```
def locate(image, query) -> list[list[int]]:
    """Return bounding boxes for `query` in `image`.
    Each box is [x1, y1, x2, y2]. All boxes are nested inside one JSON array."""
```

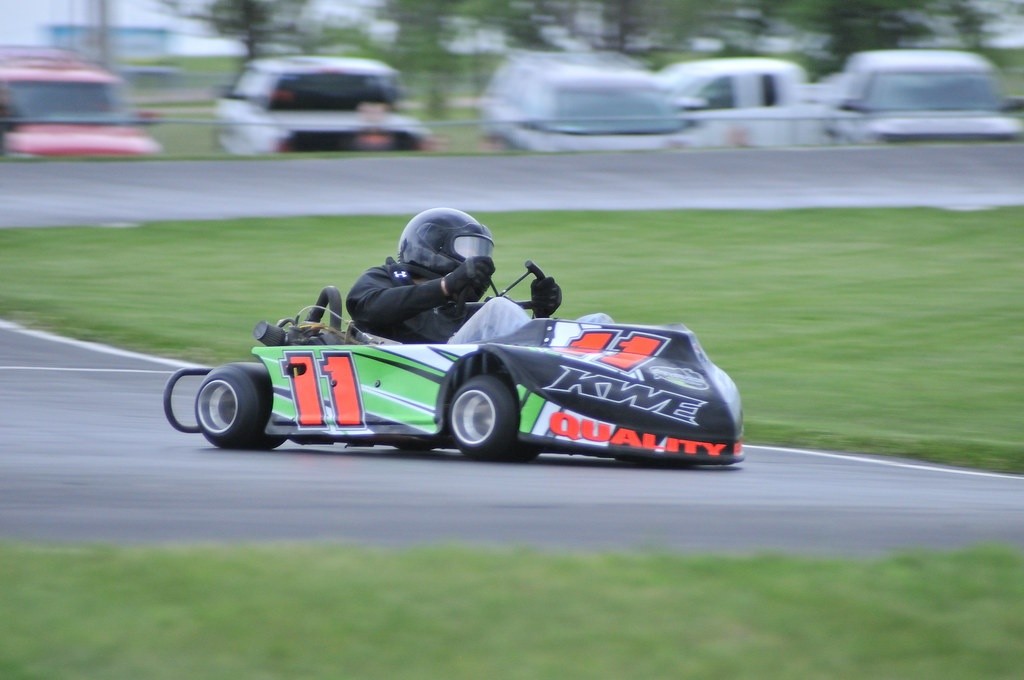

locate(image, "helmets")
[[397, 207, 495, 281]]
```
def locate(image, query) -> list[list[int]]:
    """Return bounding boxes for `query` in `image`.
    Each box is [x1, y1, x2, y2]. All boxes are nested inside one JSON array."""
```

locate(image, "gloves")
[[530, 276, 563, 317], [442, 255, 496, 295]]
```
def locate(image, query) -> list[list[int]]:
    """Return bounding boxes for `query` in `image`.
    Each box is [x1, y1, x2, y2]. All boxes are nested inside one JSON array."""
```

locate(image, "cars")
[[474, 49, 688, 152], [823, 48, 1024, 142]]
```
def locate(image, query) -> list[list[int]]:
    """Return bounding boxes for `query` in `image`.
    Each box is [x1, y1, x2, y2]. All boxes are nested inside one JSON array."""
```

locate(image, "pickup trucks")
[[657, 56, 832, 148], [211, 55, 438, 155]]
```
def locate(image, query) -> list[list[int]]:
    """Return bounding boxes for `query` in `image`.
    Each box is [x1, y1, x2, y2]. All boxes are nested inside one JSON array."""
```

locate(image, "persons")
[[346, 208, 616, 345]]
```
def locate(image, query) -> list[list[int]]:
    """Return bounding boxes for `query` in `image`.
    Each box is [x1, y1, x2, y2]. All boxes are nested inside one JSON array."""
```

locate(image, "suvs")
[[0, 45, 162, 158]]
